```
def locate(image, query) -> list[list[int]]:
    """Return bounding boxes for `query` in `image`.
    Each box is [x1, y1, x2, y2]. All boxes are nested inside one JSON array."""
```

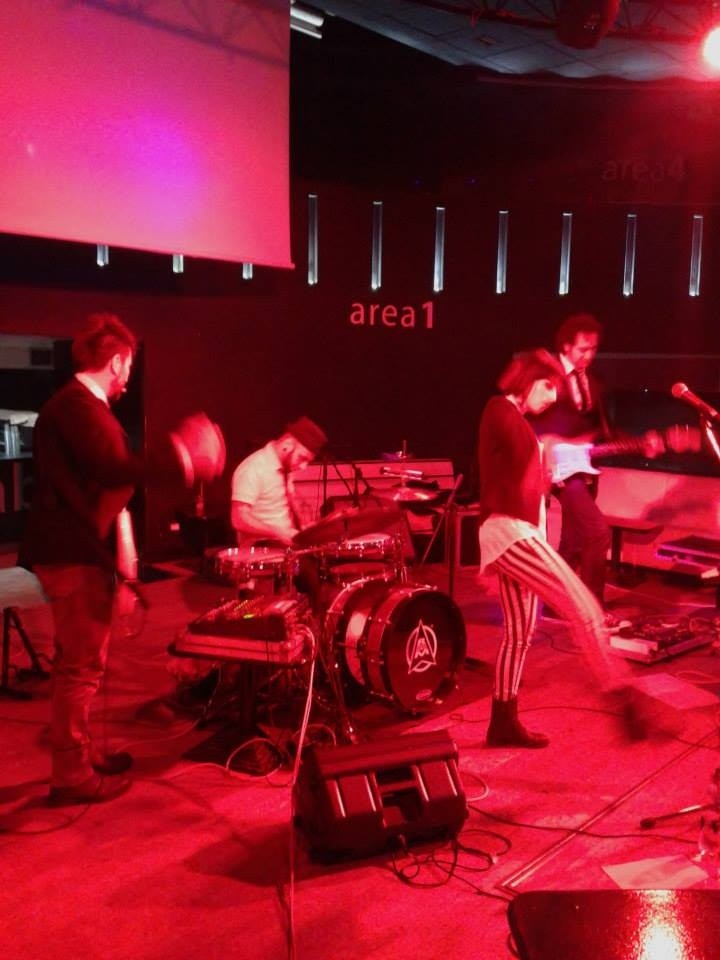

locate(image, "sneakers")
[[92, 752, 132, 776], [600, 613, 633, 633], [48, 770, 130, 805], [539, 605, 569, 625]]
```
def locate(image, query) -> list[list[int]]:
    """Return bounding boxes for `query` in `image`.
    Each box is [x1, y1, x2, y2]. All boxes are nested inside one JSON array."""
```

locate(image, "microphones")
[[671, 382, 720, 424]]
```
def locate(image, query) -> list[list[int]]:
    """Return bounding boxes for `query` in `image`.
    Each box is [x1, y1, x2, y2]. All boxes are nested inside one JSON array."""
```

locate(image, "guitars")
[[114, 510, 138, 617], [542, 424, 687, 483]]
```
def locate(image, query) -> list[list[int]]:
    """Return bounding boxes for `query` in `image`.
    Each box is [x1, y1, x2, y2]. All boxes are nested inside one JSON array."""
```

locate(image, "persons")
[[533, 311, 657, 632], [479, 348, 647, 748], [15, 311, 159, 806], [229, 414, 327, 546]]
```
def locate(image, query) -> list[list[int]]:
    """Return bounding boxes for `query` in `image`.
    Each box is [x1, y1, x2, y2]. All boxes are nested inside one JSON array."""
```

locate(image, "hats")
[[284, 415, 328, 457], [168, 409, 226, 519], [528, 346, 566, 381]]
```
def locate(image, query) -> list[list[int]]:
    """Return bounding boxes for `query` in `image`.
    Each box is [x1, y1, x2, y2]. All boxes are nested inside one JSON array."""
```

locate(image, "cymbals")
[[371, 486, 440, 501], [292, 504, 393, 540]]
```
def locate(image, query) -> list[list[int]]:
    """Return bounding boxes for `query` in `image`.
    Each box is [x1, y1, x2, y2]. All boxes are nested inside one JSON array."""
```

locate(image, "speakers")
[[292, 728, 470, 865]]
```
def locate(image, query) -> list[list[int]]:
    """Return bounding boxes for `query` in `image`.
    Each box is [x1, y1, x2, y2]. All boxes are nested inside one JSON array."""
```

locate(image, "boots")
[[616, 686, 649, 744], [485, 695, 551, 748]]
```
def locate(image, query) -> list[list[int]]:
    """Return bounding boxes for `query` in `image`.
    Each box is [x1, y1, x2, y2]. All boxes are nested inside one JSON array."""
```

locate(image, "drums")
[[316, 533, 396, 556], [325, 577, 465, 714], [327, 560, 397, 595], [216, 547, 294, 595]]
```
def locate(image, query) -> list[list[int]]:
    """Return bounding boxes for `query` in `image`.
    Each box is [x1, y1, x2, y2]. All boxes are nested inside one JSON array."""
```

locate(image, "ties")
[[280, 468, 301, 532], [572, 369, 588, 412]]
